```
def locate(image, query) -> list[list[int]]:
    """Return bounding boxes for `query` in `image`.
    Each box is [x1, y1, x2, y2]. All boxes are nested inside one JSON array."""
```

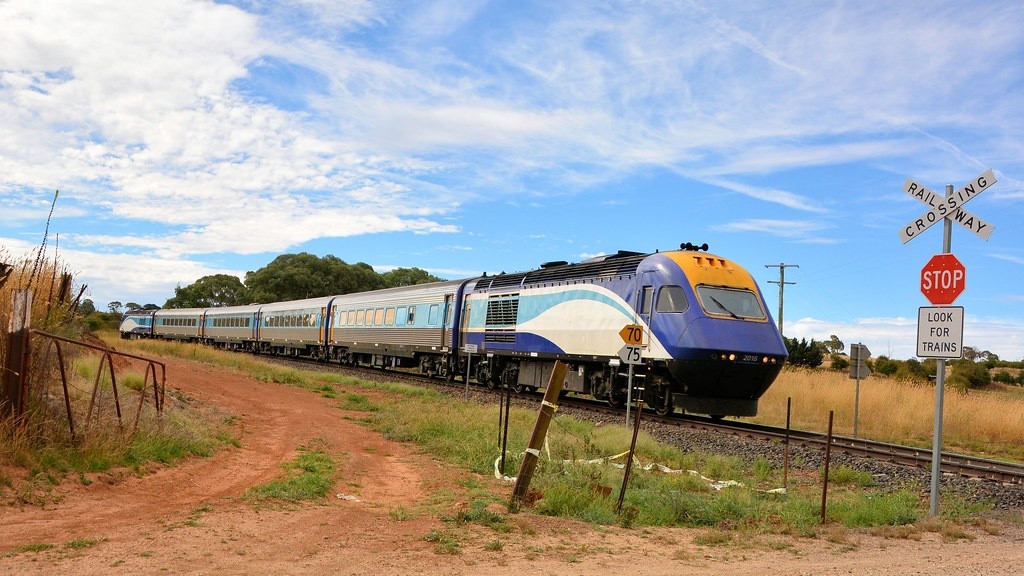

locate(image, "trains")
[[119, 242, 790, 421]]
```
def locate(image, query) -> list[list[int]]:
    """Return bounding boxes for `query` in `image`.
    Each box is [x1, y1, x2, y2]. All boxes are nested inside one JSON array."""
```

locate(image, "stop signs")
[[920, 253, 966, 305]]
